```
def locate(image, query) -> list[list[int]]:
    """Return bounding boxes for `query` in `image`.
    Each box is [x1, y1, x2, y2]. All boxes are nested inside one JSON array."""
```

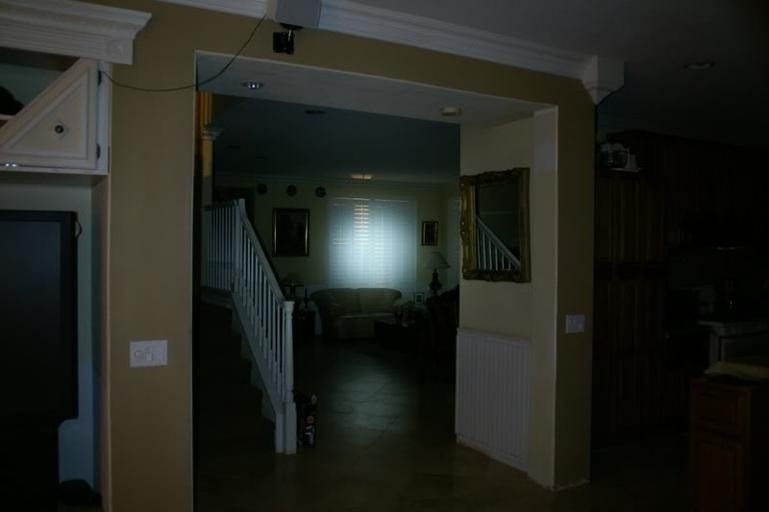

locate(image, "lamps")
[[423, 252, 450, 298]]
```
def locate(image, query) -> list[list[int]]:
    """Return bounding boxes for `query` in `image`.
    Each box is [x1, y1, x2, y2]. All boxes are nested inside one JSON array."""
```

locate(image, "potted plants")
[[294, 392, 320, 448]]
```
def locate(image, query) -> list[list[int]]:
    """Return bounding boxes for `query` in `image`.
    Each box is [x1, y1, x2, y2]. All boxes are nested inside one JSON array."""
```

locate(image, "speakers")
[[272, 1, 323, 31]]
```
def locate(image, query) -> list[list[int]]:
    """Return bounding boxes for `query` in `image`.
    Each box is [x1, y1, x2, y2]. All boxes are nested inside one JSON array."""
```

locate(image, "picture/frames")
[[272, 208, 309, 256], [422, 221, 438, 245]]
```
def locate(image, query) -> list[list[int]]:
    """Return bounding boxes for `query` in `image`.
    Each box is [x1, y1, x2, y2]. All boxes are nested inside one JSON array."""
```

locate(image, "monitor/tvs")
[[1, 207, 80, 418]]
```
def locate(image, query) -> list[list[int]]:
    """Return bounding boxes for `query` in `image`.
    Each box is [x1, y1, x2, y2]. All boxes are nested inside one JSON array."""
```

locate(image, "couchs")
[[311, 288, 401, 344]]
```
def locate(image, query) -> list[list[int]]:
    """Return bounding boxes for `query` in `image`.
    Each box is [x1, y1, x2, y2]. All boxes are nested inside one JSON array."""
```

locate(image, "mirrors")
[[459, 168, 530, 283], [0, 210, 78, 512]]
